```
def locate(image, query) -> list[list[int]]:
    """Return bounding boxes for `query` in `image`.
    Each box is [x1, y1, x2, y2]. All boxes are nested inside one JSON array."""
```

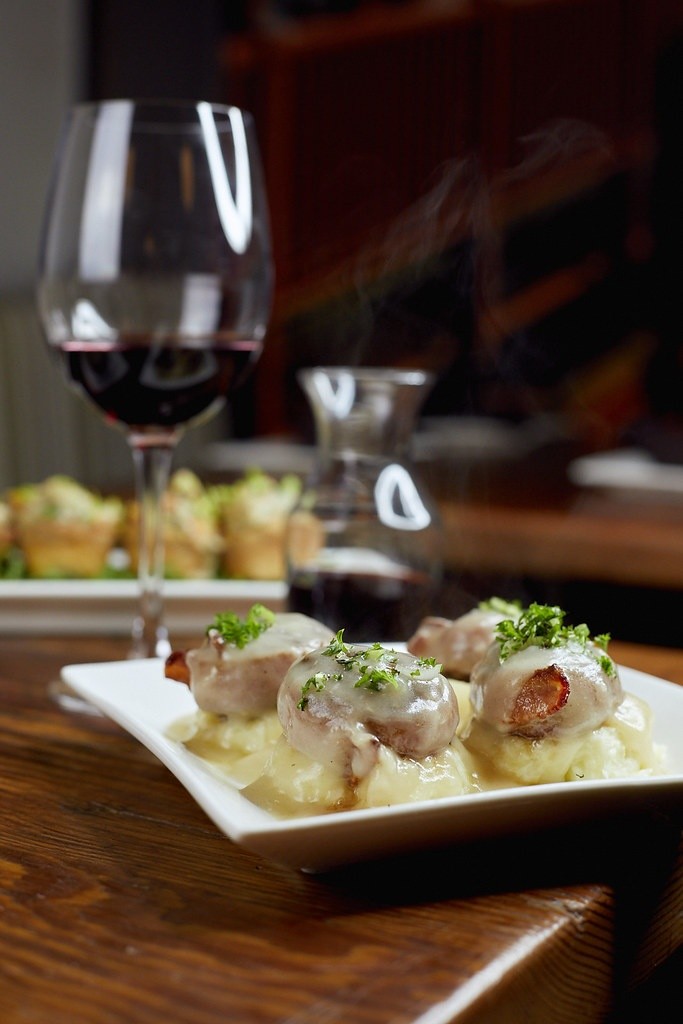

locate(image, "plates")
[[0, 554, 285, 632], [59, 635, 682, 854]]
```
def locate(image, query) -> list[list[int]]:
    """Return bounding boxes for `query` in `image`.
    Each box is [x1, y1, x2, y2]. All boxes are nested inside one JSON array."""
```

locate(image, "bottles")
[[287, 366, 439, 633]]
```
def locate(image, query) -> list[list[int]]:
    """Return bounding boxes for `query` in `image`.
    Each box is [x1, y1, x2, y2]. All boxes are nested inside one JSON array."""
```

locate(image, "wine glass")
[[33, 93, 273, 725]]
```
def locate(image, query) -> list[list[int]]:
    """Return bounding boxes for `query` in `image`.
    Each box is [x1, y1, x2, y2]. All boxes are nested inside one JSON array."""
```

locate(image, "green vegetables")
[[484, 598, 614, 678], [298, 628, 440, 711], [205, 605, 275, 645]]
